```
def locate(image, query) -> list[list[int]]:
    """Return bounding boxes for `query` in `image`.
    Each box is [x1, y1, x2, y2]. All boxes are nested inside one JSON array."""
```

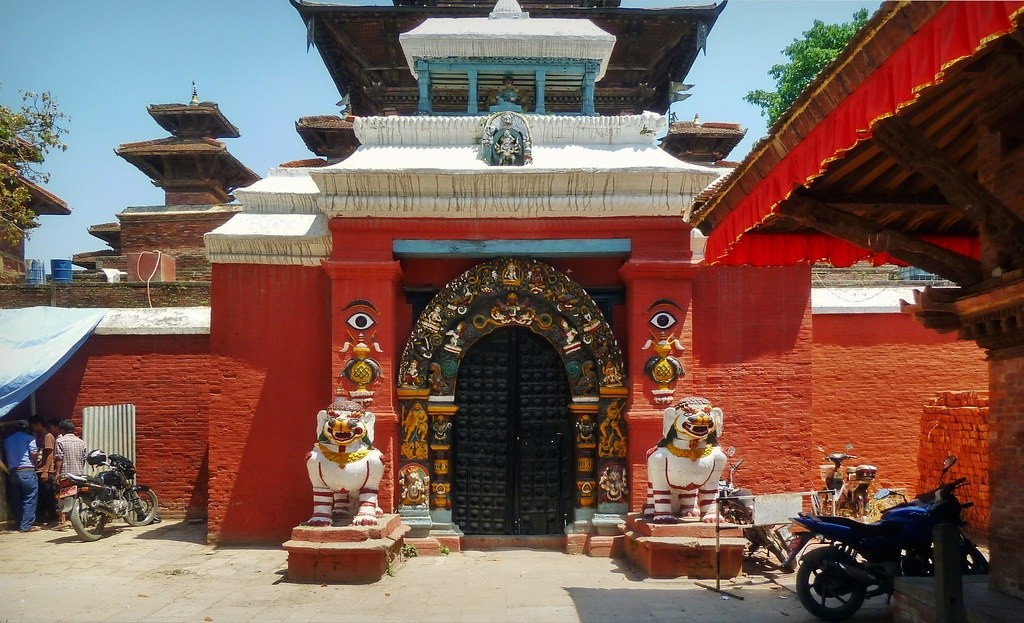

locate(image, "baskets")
[[819, 465, 843, 481]]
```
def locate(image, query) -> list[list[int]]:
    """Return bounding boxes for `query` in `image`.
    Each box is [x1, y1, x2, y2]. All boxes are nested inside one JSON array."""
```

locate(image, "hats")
[[16, 420, 29, 428]]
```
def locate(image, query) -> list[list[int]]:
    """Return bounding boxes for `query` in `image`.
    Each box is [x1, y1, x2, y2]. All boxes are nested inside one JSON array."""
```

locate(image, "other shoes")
[[31, 522, 39, 526], [52, 524, 67, 530], [20, 526, 40, 533]]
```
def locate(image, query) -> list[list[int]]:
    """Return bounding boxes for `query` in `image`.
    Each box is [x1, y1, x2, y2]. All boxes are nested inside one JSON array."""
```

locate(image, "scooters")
[[818, 443, 878, 519]]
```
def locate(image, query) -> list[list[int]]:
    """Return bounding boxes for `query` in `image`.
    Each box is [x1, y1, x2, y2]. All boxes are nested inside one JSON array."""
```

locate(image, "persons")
[[0, 413, 87, 533]]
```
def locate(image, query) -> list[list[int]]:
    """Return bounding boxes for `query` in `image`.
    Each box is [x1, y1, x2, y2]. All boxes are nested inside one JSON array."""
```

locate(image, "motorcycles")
[[59, 449, 159, 542], [719, 445, 798, 572], [792, 455, 990, 623]]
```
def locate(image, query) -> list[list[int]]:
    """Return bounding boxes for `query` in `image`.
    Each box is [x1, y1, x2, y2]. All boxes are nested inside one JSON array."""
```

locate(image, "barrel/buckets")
[[51, 259, 72, 283], [24, 259, 47, 283]]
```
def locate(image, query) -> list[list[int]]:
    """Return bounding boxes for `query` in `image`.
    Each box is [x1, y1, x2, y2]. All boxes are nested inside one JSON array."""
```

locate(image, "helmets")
[[88, 449, 106, 465]]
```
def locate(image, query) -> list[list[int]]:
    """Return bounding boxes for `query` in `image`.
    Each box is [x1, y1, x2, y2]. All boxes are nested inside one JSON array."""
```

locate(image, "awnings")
[[0, 306, 110, 419]]
